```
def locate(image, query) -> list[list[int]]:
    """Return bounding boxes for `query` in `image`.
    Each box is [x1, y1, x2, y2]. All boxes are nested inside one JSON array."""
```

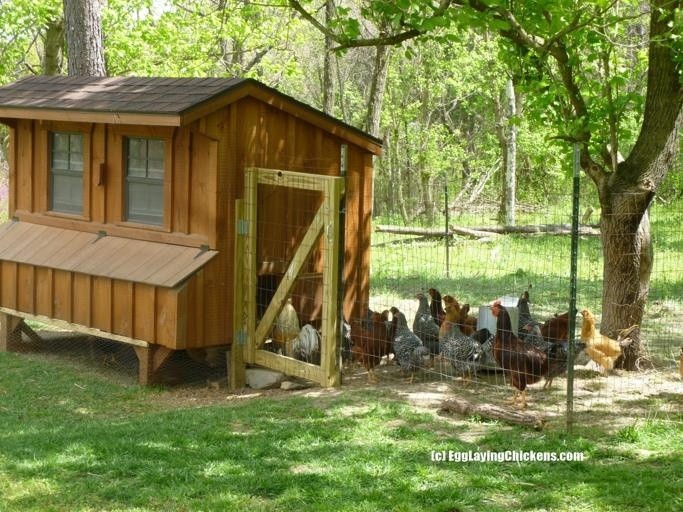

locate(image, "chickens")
[[340, 287, 633, 411]]
